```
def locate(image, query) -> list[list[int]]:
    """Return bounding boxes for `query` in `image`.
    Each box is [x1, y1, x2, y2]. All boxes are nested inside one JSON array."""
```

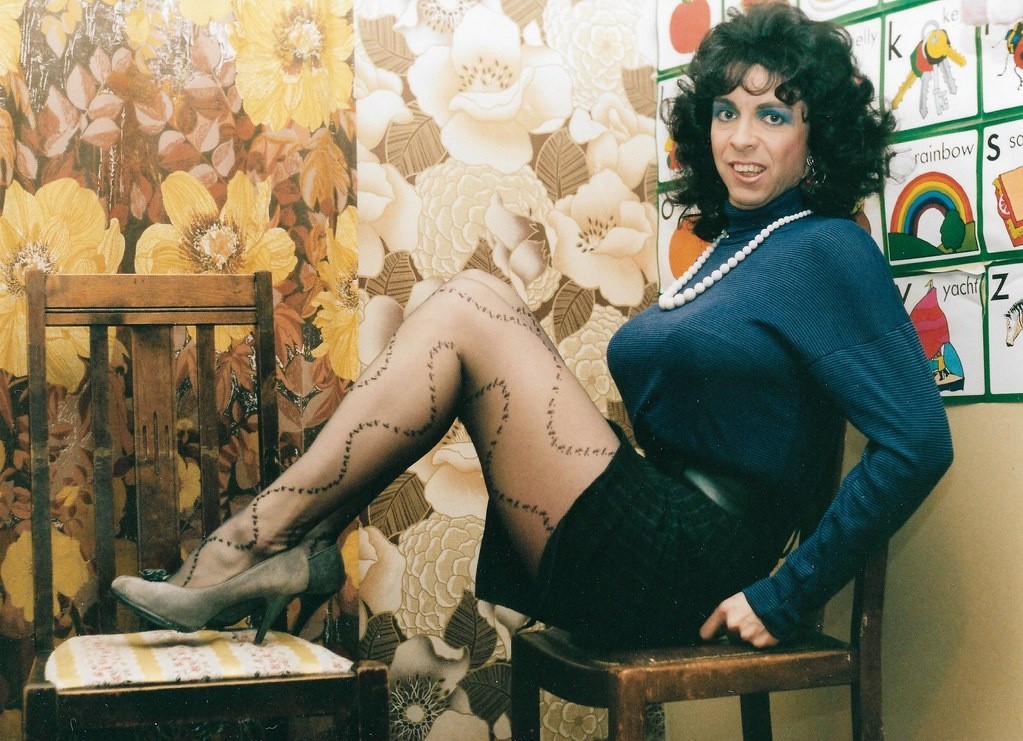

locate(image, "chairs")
[[21, 267, 391, 741], [509, 538, 890, 741]]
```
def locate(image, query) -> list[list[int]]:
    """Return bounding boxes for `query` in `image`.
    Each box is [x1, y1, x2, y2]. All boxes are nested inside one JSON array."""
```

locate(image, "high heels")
[[110, 546, 309, 645], [207, 544, 346, 637]]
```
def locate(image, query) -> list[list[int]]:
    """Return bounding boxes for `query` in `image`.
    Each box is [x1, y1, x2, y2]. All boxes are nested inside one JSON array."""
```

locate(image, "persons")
[[111, 0, 954, 648]]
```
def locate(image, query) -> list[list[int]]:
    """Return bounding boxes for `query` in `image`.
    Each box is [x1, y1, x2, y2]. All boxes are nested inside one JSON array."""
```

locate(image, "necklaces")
[[659, 210, 812, 309]]
